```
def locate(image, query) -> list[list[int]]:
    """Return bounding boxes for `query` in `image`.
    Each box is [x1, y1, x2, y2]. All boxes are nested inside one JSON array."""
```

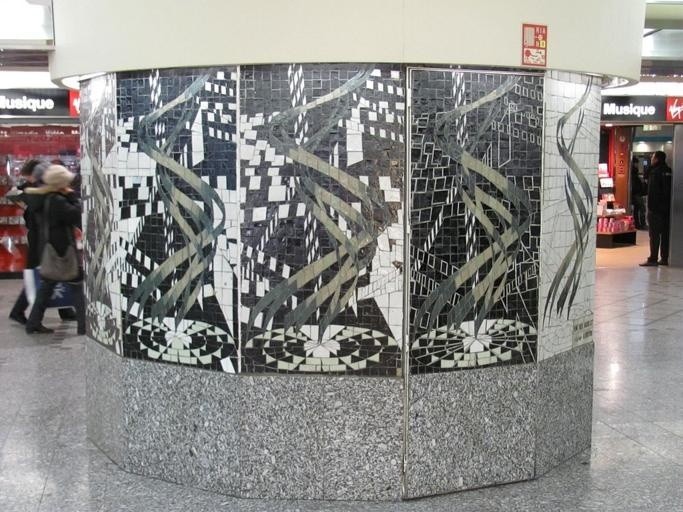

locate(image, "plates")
[[596, 177, 636, 248]]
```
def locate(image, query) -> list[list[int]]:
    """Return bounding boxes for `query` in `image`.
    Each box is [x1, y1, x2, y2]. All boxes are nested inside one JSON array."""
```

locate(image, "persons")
[[9, 160, 83, 334], [631, 155, 647, 230], [639, 151, 673, 266]]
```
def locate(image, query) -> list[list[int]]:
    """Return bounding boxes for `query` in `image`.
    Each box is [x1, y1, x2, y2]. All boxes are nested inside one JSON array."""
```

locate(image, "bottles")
[[41, 164, 81, 188]]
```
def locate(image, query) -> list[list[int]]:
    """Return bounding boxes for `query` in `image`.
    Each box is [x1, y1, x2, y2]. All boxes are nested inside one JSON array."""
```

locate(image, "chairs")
[[639, 256, 669, 267], [9, 309, 27, 325], [24, 323, 55, 334]]
